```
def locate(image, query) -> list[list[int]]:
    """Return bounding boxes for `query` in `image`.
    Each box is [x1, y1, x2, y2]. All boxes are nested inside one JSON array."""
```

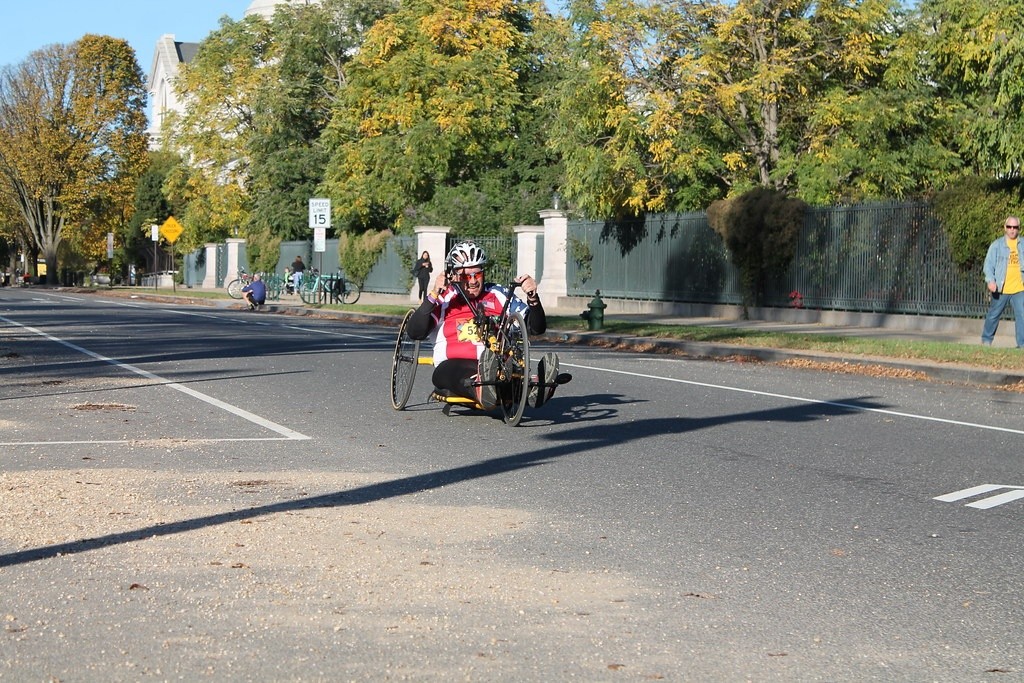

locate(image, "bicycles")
[[227, 266, 282, 301], [298, 266, 362, 305]]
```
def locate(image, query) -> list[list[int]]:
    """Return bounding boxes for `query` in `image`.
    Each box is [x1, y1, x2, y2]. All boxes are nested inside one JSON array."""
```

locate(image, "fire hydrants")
[[577, 288, 608, 331]]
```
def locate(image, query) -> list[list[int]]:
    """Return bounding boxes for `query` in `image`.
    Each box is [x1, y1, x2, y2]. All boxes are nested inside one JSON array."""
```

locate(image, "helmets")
[[444, 240, 487, 269]]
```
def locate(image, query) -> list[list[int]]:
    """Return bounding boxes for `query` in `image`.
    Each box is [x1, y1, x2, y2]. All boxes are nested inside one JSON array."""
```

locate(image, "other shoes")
[[244, 304, 254, 311], [475, 349, 498, 410], [253, 303, 260, 312], [528, 352, 560, 408]]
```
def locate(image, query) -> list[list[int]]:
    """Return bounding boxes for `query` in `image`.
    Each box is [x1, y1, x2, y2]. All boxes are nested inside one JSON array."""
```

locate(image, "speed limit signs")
[[308, 197, 331, 230]]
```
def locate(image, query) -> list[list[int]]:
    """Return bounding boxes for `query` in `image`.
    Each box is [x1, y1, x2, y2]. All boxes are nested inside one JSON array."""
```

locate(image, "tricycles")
[[389, 260, 572, 427]]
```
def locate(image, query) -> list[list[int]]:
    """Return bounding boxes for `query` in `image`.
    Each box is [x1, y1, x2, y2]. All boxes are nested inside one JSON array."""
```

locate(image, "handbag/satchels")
[[410, 260, 421, 277]]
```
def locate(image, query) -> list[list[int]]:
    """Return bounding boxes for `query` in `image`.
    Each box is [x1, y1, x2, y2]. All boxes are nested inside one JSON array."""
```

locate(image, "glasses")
[[1006, 225, 1019, 230], [453, 268, 484, 282]]
